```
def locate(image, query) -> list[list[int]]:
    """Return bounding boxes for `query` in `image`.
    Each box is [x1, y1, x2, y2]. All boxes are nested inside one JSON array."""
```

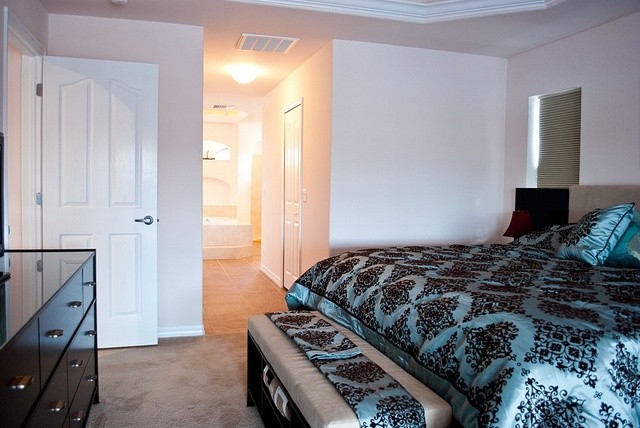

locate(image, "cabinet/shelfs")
[[0, 249, 100, 428]]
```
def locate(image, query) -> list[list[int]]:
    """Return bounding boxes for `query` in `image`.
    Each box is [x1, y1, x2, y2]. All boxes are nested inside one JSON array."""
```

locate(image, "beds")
[[287, 186, 640, 428]]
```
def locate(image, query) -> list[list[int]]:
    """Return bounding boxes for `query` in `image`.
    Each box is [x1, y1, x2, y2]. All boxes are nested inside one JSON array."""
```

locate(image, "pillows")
[[557, 202, 635, 265], [514, 223, 575, 255], [604, 219, 639, 265]]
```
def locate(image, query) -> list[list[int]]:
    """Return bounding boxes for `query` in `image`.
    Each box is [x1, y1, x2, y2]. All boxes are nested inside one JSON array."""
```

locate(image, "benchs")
[[248, 308, 451, 427]]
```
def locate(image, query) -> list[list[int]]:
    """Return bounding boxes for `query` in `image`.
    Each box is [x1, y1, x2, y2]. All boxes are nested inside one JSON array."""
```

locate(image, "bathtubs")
[[203, 217, 253, 259]]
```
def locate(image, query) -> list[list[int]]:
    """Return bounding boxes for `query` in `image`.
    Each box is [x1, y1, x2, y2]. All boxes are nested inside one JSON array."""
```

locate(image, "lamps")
[[504, 210, 534, 242], [227, 63, 262, 86]]
